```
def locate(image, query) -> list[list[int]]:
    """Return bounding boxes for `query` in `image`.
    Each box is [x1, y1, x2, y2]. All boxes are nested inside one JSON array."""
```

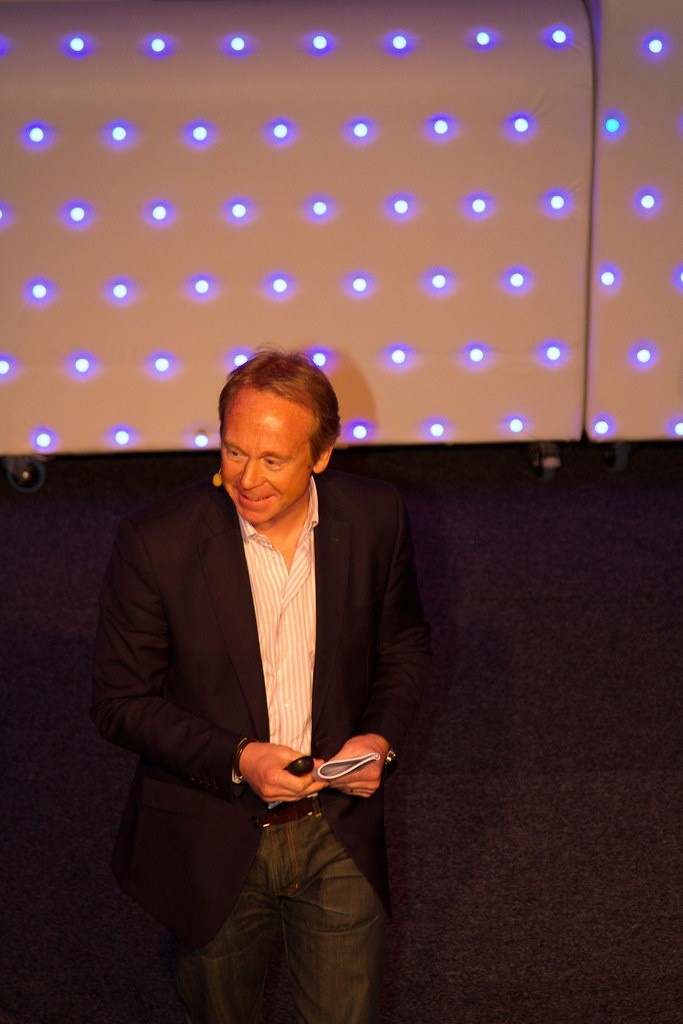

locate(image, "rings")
[[350, 788, 353, 793]]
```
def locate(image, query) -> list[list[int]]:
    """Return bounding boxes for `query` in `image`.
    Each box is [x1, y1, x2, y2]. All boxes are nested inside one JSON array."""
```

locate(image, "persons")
[[91, 353, 427, 1024]]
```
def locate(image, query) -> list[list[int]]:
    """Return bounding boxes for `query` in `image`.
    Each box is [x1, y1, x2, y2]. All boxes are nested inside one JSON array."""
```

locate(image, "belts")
[[261, 794, 320, 827]]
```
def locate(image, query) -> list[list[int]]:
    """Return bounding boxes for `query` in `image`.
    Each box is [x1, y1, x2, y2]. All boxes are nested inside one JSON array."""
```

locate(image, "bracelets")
[[234, 738, 259, 782]]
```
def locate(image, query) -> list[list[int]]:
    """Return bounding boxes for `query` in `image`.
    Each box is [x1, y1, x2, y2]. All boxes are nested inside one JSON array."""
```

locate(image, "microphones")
[[213, 469, 223, 487]]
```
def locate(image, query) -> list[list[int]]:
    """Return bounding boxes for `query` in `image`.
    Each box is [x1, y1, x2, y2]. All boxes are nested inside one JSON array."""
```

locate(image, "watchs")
[[384, 748, 396, 766]]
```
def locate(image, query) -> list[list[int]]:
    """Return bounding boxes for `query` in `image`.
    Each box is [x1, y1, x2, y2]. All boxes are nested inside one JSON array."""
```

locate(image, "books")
[[313, 753, 378, 781]]
